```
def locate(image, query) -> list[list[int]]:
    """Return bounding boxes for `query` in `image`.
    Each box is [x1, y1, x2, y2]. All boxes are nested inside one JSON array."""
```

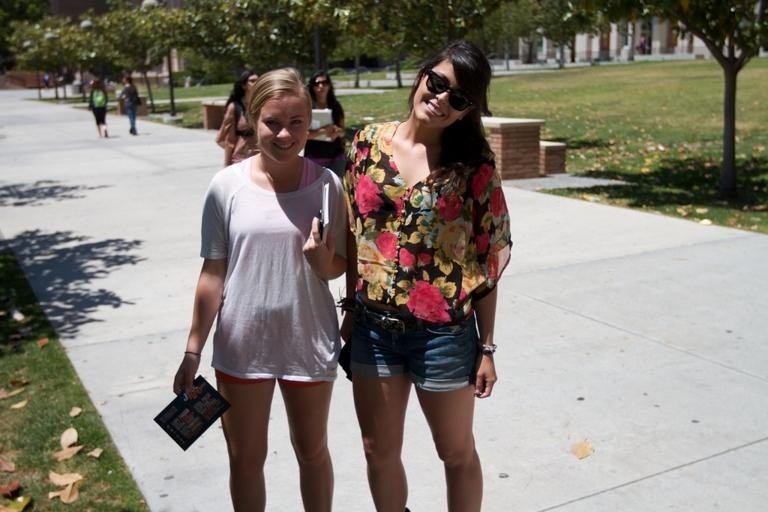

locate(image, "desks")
[[480, 116, 545, 181], [117, 96, 147, 116], [201, 101, 228, 129]]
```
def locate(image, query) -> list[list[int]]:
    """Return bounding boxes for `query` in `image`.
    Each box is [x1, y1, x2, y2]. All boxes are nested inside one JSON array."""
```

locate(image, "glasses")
[[422, 66, 476, 111], [313, 79, 329, 87]]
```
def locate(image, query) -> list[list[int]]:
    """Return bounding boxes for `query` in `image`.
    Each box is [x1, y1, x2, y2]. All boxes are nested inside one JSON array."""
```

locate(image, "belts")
[[352, 298, 417, 334]]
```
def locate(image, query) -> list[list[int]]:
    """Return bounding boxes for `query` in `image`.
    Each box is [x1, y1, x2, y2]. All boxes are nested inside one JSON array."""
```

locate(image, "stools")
[[540, 141, 567, 175]]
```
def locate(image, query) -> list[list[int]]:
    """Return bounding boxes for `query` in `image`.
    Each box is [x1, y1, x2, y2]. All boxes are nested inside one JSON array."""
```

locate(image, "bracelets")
[[183, 350, 203, 357], [477, 340, 497, 355], [337, 297, 357, 314]]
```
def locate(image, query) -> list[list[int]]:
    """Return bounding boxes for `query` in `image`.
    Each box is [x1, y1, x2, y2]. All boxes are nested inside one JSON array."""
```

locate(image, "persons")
[[88, 79, 108, 138], [335, 38, 514, 511], [116, 75, 138, 135], [639, 33, 647, 55], [303, 70, 349, 181], [171, 66, 351, 510], [214, 68, 263, 171]]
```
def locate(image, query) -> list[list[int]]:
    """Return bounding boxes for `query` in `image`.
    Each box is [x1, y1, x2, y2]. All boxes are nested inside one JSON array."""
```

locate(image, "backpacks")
[[93, 89, 107, 108], [128, 89, 141, 106]]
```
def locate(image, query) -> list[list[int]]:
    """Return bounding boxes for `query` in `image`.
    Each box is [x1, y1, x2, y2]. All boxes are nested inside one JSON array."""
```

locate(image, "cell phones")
[[319, 208, 324, 240]]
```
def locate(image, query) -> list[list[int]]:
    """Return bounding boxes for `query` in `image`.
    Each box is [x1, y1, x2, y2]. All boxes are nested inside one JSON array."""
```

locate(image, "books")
[[320, 180, 332, 245], [308, 108, 332, 143]]
